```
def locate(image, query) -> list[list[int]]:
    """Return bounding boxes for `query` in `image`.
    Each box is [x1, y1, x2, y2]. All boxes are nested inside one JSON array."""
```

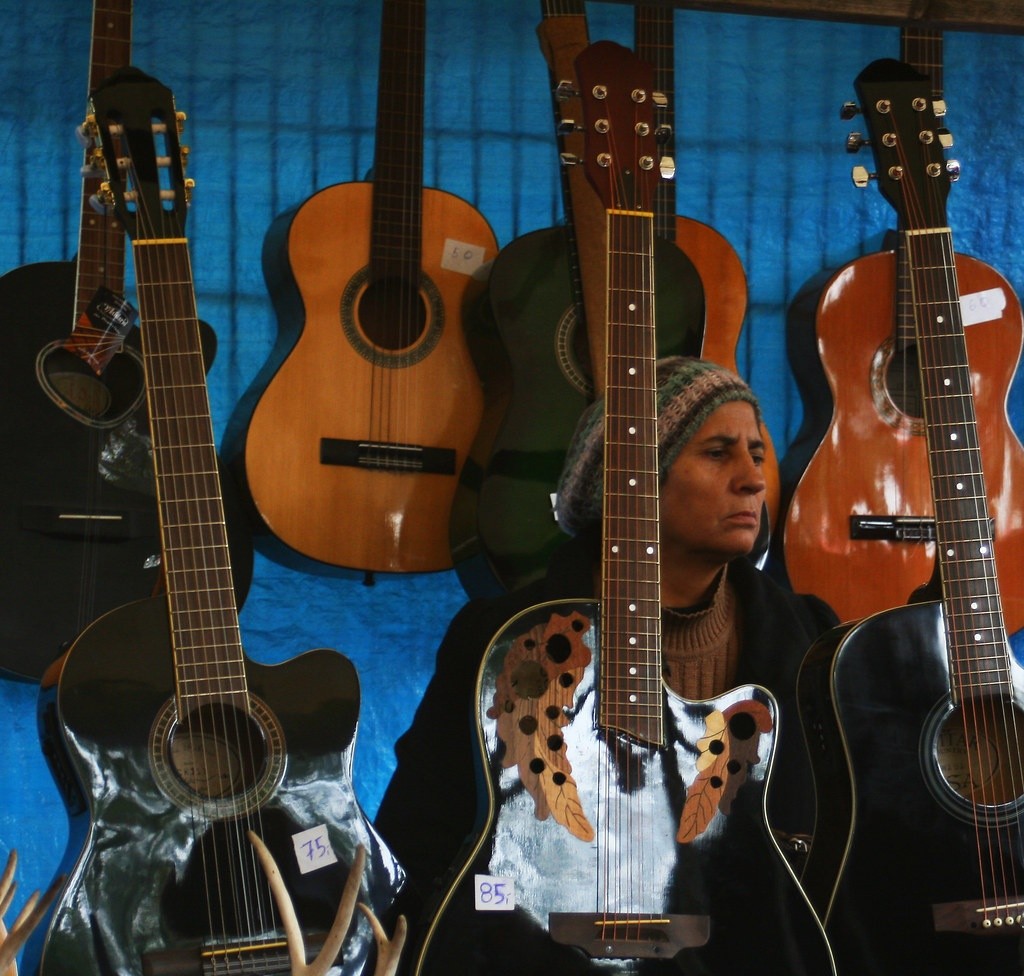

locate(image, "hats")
[[555, 356, 761, 537]]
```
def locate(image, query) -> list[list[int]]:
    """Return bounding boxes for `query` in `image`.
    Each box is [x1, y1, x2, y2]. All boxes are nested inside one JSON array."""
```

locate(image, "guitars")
[[792, 56, 1024, 975], [373, 42, 841, 976], [768, 1, 1024, 641], [635, 1, 782, 545], [0, 0, 255, 687], [219, 2, 506, 585], [20, 64, 371, 976], [445, 1, 710, 599]]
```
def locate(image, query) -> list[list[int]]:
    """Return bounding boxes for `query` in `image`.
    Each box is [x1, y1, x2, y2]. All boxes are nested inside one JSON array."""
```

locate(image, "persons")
[[372, 357, 839, 976]]
